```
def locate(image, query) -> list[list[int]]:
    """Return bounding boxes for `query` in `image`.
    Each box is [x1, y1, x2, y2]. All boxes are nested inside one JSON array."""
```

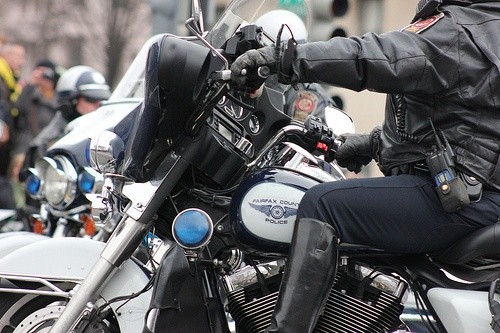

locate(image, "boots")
[[263, 218, 341, 333]]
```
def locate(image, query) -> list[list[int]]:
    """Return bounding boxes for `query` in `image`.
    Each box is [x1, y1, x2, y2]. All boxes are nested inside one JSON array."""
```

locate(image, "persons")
[[230, 0, 500, 333], [0, 52, 112, 232]]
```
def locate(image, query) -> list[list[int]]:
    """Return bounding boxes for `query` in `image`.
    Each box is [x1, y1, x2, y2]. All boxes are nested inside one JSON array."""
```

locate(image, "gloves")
[[230, 40, 284, 94], [323, 127, 378, 174]]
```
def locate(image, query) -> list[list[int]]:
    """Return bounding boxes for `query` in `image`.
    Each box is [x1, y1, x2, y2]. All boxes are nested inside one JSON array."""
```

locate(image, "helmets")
[[56, 64, 110, 106]]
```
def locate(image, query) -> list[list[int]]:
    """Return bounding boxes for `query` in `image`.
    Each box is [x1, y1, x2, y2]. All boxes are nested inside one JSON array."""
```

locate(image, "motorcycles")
[[1, 0, 499, 333]]
